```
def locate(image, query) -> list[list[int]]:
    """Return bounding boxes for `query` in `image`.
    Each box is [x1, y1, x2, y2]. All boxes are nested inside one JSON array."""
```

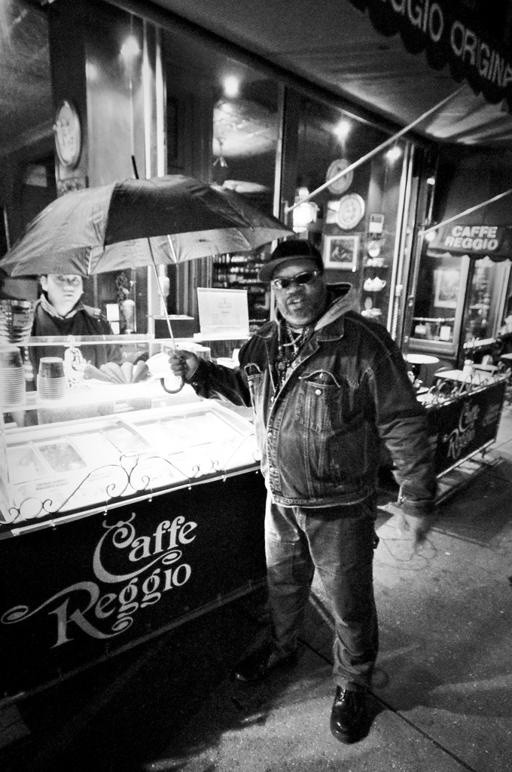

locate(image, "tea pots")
[[364, 276, 386, 291]]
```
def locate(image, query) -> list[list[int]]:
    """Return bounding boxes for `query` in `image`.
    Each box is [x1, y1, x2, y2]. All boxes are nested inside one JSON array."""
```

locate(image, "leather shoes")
[[330, 684, 363, 744], [231, 641, 299, 685]]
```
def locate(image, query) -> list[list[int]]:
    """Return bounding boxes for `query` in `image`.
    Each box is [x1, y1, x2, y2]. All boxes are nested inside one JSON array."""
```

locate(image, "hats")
[[257, 239, 322, 280]]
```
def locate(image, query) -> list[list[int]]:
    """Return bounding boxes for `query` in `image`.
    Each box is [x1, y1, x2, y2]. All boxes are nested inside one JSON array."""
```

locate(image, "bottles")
[[462, 359, 474, 380], [1, 299, 33, 346]]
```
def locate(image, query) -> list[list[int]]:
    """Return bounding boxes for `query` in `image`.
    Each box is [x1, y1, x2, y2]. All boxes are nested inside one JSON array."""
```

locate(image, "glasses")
[[270, 270, 323, 289]]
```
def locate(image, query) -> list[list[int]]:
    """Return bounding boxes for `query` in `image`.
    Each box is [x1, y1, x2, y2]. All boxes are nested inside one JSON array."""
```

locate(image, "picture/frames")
[[321, 234, 360, 273]]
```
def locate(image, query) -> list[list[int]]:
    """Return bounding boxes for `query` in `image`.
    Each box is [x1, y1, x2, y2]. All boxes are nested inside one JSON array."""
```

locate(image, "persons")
[[165, 237, 436, 744], [32, 270, 121, 370]]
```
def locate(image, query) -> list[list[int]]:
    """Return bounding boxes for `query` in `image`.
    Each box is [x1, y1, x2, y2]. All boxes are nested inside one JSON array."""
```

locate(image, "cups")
[[439, 326, 451, 341], [415, 325, 426, 339], [1, 347, 65, 406]]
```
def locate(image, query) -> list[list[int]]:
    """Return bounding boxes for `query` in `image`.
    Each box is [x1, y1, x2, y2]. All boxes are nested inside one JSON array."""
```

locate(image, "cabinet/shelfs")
[[0, 332, 269, 771], [205, 237, 273, 328]]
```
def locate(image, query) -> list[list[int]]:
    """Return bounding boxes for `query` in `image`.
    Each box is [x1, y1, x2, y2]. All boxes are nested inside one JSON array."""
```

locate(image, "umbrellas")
[[1, 152, 297, 394]]
